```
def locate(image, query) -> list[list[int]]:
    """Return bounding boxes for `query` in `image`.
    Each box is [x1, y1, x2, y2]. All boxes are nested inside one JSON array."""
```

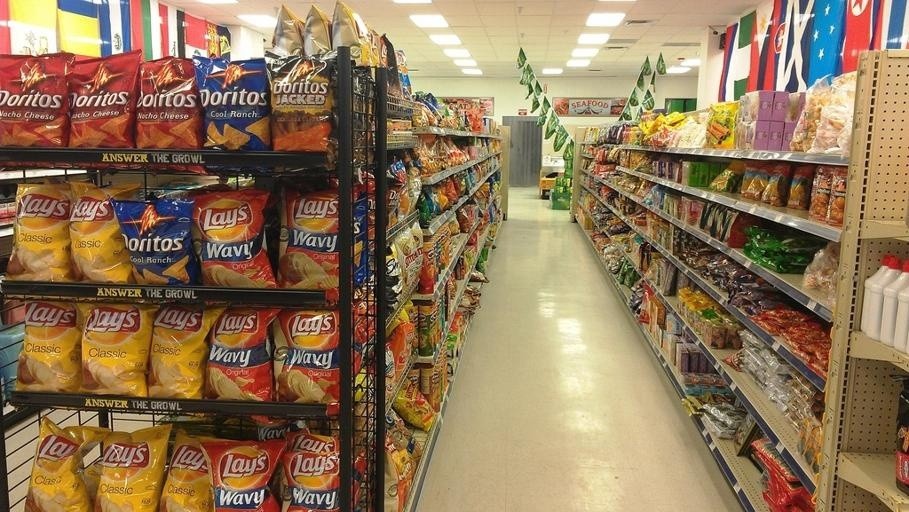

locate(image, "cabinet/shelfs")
[[540, 154, 565, 200], [380, 101, 506, 511], [816, 51, 905, 512], [575, 55, 853, 512], [0, 50, 385, 512]]
